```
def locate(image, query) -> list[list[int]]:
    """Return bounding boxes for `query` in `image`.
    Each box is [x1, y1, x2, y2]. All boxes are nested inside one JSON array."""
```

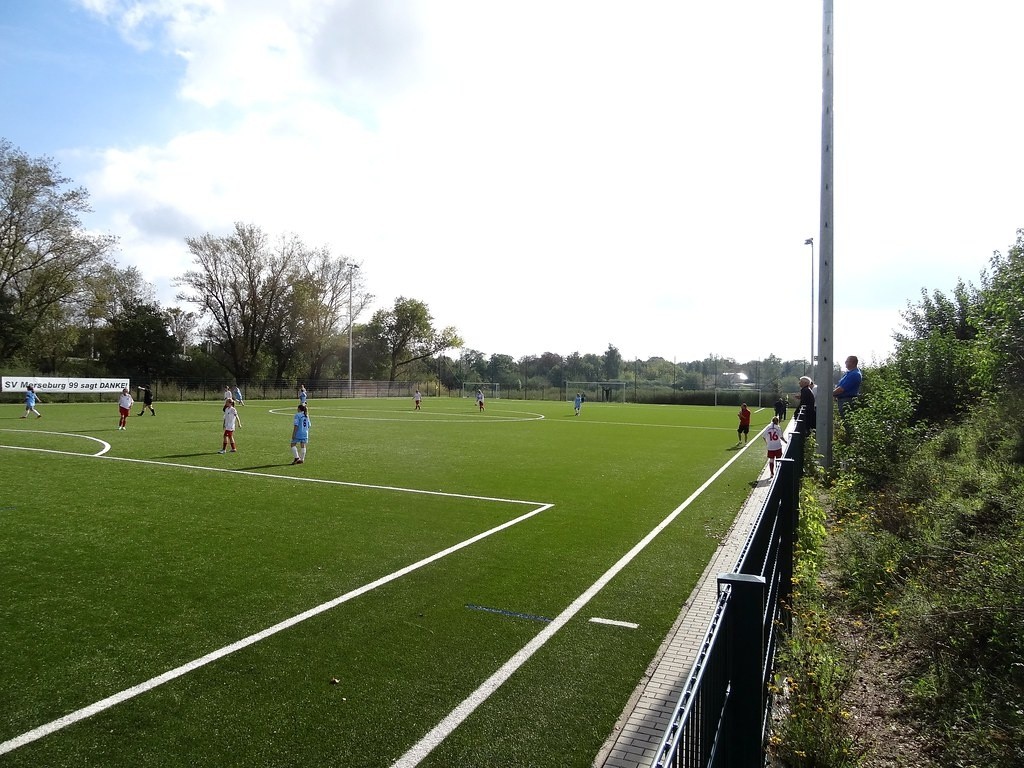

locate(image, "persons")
[[475, 389, 485, 413], [298, 385, 308, 407], [581, 392, 585, 402], [794, 376, 817, 437], [118, 388, 134, 431], [137, 385, 156, 416], [291, 405, 311, 465], [574, 393, 581, 416], [412, 389, 422, 410], [603, 388, 610, 403], [19, 386, 42, 419], [833, 356, 862, 418], [218, 399, 241, 454], [762, 416, 787, 479], [222, 385, 244, 412], [773, 397, 789, 423], [736, 403, 751, 446]]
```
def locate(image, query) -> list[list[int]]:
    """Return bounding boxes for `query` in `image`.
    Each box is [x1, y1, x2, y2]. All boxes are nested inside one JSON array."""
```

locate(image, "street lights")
[[805, 237, 814, 382], [347, 263, 359, 395]]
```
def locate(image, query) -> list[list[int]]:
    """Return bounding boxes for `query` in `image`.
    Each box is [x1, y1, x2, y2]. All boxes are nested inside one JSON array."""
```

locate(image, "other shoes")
[[37, 415, 41, 418], [137, 414, 143, 416], [20, 416, 26, 418], [151, 414, 156, 416], [118, 426, 126, 430], [736, 442, 747, 447], [292, 458, 303, 464]]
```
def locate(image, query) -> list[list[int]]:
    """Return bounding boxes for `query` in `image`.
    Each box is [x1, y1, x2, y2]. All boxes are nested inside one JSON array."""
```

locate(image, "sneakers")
[[229, 449, 236, 452], [218, 449, 226, 454]]
